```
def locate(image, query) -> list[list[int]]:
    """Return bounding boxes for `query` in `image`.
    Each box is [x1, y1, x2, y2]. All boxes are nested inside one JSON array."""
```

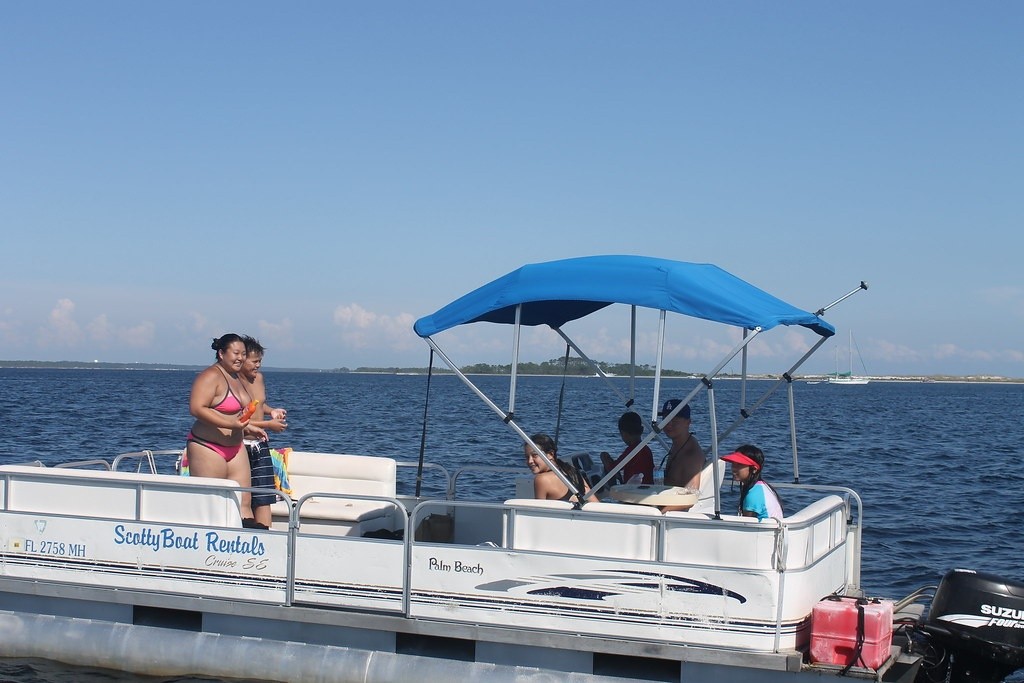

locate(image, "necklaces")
[[664, 433, 693, 478]]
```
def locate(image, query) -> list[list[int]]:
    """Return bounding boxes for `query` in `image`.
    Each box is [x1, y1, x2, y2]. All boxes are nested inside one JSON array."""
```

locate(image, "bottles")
[[237, 400, 259, 422], [653, 464, 665, 490], [627, 473, 644, 490]]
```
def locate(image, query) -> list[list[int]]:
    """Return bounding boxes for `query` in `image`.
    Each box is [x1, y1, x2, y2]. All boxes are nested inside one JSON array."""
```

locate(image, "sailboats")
[[826, 329, 870, 385]]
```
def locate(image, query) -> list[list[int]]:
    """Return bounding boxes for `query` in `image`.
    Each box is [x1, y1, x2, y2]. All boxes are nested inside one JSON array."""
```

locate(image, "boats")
[[806, 381, 821, 385], [0, 255, 1024, 683], [595, 372, 617, 378]]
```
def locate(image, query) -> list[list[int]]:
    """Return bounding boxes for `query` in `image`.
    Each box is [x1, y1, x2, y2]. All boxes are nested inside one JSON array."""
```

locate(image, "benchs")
[[271, 451, 397, 537]]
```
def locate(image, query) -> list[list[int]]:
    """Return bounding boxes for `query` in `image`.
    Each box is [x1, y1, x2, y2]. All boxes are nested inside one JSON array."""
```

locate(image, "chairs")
[[681, 458, 726, 513]]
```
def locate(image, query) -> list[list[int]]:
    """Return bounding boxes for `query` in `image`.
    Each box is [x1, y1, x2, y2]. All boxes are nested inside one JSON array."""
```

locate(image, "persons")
[[608, 412, 654, 485], [187, 334, 288, 530], [525, 433, 600, 502], [719, 445, 783, 521], [658, 399, 705, 514]]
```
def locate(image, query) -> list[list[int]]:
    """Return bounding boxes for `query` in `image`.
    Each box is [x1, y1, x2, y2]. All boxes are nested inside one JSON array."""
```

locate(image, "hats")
[[719, 451, 760, 471], [657, 399, 690, 418]]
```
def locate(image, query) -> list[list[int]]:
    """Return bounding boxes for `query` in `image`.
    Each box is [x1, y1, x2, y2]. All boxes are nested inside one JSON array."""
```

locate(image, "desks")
[[608, 482, 698, 509]]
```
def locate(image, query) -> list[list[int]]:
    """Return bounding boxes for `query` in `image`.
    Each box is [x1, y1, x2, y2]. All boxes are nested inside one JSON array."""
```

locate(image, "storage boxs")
[[809, 598, 895, 669]]
